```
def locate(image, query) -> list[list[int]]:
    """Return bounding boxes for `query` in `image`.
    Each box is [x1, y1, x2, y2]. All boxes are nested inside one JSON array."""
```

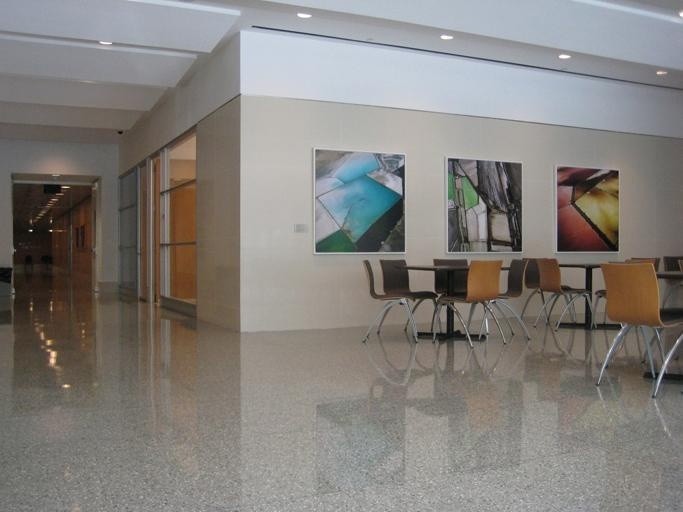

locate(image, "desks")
[[397, 261, 513, 343], [554, 259, 620, 332], [633, 266, 681, 383]]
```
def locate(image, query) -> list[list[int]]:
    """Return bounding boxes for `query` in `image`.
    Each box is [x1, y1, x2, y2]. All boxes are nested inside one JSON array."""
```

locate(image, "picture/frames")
[[444, 155, 523, 255], [553, 164, 620, 254], [313, 147, 407, 255]]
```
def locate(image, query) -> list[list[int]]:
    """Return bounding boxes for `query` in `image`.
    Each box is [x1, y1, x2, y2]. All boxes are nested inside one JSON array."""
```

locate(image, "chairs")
[[430, 259, 476, 339], [363, 260, 440, 348], [533, 258, 597, 336], [478, 259, 535, 343], [453, 259, 508, 350], [592, 262, 680, 398], [521, 258, 572, 326], [377, 259, 440, 339], [660, 258, 681, 312]]
